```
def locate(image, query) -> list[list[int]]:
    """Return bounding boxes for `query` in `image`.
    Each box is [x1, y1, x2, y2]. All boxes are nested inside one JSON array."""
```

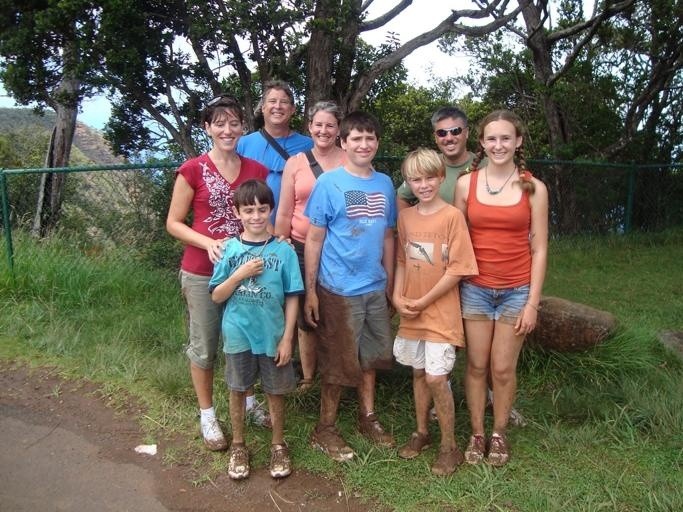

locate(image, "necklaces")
[[239, 234, 269, 257], [485, 161, 517, 195]]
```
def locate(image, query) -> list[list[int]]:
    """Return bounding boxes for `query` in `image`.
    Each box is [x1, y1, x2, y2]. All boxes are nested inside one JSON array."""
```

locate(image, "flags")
[[344, 189, 387, 220]]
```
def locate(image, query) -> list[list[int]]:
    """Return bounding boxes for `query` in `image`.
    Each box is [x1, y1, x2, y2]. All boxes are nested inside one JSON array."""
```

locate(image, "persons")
[[392, 146, 480, 475], [272, 103, 374, 391], [209, 179, 304, 483], [452, 110, 549, 466], [390, 104, 527, 429], [299, 110, 395, 464], [236, 81, 315, 224], [163, 91, 274, 453]]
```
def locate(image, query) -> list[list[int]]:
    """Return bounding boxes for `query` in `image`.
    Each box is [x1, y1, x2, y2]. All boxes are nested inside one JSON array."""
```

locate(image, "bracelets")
[[527, 302, 543, 313]]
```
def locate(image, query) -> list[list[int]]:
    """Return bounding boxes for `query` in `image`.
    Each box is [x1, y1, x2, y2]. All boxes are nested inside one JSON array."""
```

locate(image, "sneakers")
[[269, 439, 292, 478], [201, 416, 228, 451], [228, 444, 250, 480], [245, 399, 273, 431], [398, 432, 434, 460], [431, 434, 508, 476], [311, 428, 355, 462], [487, 391, 527, 427], [357, 415, 396, 449]]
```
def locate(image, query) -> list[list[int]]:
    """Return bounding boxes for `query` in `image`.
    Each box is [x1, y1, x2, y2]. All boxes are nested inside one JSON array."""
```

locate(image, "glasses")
[[434, 125, 466, 138]]
